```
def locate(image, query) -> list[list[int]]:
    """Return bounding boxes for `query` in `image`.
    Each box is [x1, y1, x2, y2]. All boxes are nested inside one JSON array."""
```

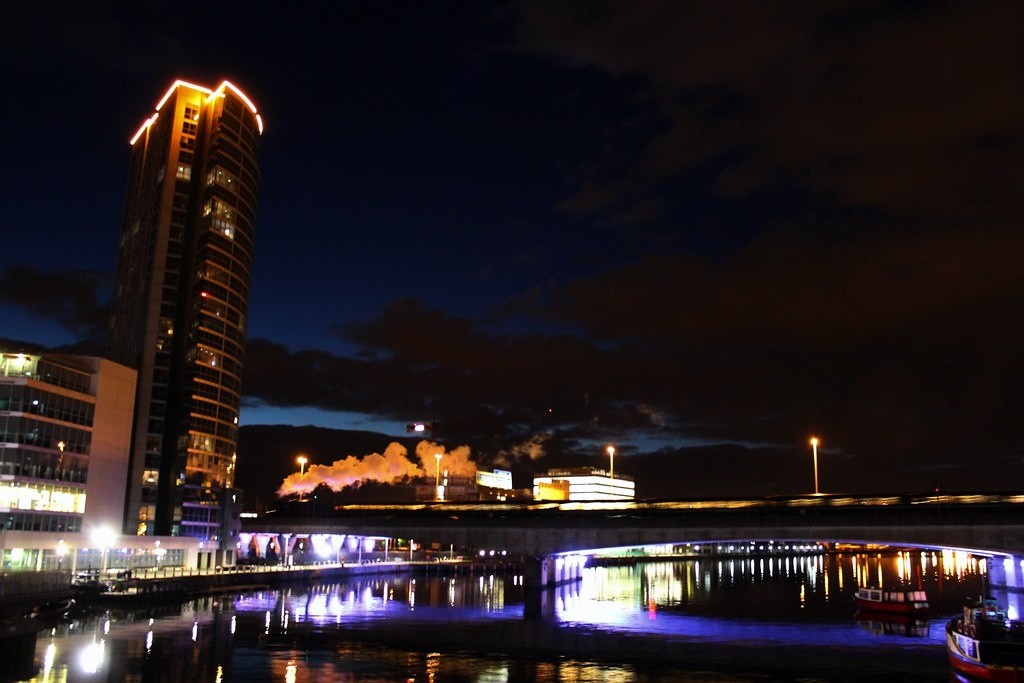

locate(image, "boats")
[[0, 598, 86, 640], [854, 583, 931, 615], [943, 596, 1024, 683]]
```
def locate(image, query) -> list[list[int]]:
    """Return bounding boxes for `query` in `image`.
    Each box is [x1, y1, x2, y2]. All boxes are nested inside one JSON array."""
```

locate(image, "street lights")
[[607, 446, 616, 478], [434, 453, 444, 501], [297, 454, 307, 505], [810, 437, 820, 494]]
